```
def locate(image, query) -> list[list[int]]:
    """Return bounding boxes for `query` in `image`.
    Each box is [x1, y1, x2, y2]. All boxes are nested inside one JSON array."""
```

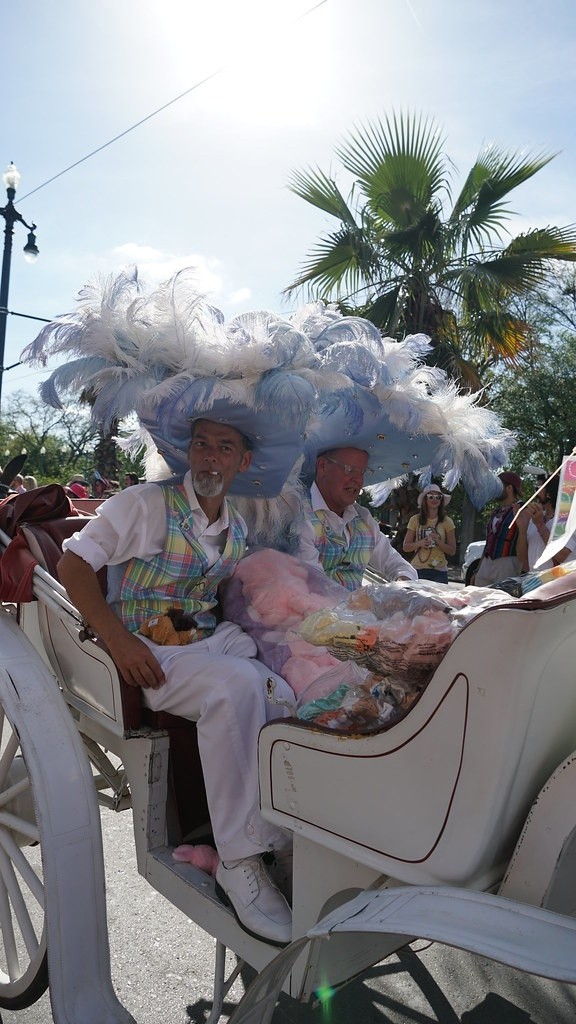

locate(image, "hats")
[[417, 485, 451, 509], [66, 474, 90, 487], [498, 472, 523, 497], [65, 483, 89, 498]]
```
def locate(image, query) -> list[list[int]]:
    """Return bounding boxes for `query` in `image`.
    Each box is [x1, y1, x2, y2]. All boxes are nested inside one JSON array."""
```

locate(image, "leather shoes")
[[215, 853, 292, 946]]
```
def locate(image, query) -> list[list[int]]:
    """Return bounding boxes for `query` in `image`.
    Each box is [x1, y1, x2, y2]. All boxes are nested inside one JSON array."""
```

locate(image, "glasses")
[[324, 455, 375, 482], [536, 486, 541, 492], [427, 494, 442, 500]]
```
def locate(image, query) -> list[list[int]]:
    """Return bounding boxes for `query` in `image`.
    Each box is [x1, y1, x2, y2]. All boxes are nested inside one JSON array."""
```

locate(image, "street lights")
[[1, 161, 40, 415]]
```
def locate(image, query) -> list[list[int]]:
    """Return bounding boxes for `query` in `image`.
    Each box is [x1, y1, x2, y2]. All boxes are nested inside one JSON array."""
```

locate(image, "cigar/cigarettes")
[[210, 471, 219, 475]]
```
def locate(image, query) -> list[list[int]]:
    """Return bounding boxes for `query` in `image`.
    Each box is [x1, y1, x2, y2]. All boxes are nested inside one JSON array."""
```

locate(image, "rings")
[[533, 512, 536, 514]]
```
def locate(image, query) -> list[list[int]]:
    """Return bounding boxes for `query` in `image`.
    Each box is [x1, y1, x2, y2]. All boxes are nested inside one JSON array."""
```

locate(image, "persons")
[[291, 447, 417, 596], [96, 477, 111, 498], [403, 485, 456, 584], [470, 472, 526, 586], [63, 474, 93, 499], [11, 474, 37, 494], [511, 472, 576, 573], [124, 472, 139, 487], [56, 416, 296, 947]]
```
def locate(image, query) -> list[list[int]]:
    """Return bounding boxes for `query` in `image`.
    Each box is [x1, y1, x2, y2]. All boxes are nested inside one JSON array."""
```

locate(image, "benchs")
[[259, 568, 576, 891], [18, 515, 197, 739]]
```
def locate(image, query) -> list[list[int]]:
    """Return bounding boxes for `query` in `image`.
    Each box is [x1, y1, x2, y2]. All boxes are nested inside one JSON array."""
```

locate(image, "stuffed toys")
[[140, 607, 217, 647], [172, 844, 220, 878], [221, 547, 566, 736]]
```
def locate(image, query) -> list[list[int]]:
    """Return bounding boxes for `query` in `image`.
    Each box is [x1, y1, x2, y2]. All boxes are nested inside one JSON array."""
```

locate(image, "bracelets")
[[537, 522, 549, 536]]
